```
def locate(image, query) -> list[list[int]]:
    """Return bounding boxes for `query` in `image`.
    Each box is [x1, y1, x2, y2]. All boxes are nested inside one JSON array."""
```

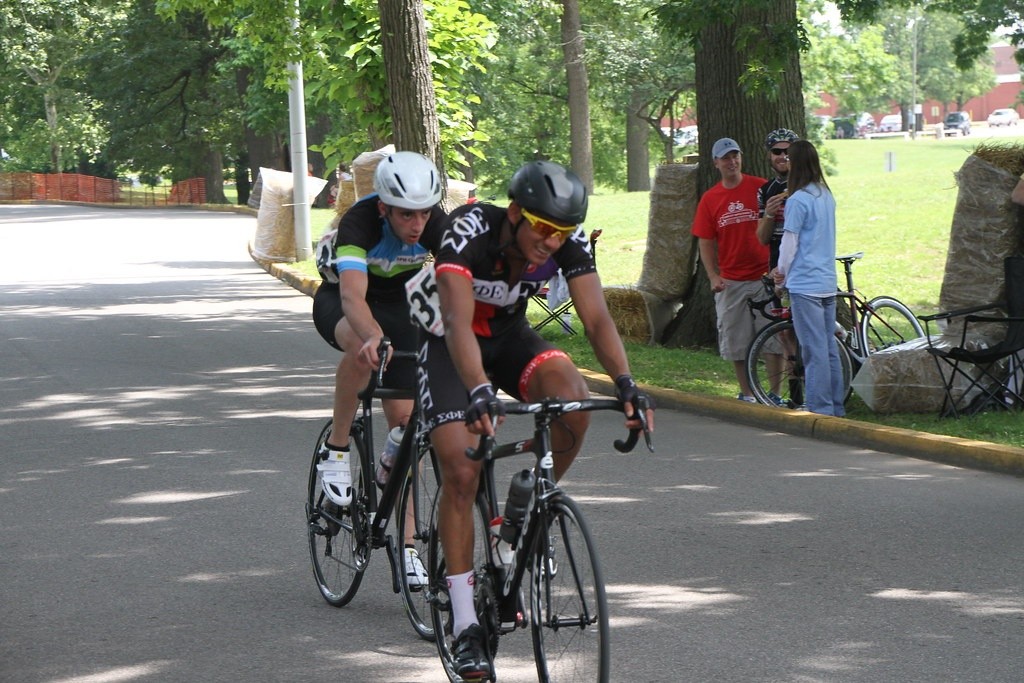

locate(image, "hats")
[[713, 139, 744, 159]]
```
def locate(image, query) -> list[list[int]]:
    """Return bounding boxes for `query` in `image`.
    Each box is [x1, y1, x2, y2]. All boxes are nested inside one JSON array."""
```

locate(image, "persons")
[[690, 129, 846, 418], [313, 151, 448, 586], [417, 161, 654, 679]]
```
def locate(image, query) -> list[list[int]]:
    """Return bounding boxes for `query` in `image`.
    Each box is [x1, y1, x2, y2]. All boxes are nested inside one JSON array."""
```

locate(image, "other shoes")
[[768, 393, 782, 407], [738, 392, 756, 404]]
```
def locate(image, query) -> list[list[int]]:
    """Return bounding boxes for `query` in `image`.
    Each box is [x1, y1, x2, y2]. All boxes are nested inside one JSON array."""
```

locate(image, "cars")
[[812, 112, 916, 139], [943, 111, 971, 137], [987, 108, 1020, 127], [661, 125, 698, 147]]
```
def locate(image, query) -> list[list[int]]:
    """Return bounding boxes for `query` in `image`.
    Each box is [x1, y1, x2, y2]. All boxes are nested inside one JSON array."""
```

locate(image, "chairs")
[[529, 228, 603, 335], [916, 256, 1024, 423]]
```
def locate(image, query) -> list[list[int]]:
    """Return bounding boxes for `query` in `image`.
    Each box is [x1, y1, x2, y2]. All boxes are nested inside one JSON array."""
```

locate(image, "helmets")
[[765, 128, 799, 149], [374, 150, 442, 210], [507, 160, 587, 224]]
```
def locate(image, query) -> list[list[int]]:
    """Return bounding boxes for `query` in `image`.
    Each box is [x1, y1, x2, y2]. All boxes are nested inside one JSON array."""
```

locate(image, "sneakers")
[[451, 623, 490, 679], [404, 547, 429, 585], [536, 534, 558, 579], [316, 430, 353, 505]]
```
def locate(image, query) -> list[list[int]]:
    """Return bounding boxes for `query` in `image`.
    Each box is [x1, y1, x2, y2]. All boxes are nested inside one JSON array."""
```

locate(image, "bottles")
[[500, 468, 536, 545], [490, 515, 515, 569], [376, 420, 405, 485], [834, 320, 848, 340]]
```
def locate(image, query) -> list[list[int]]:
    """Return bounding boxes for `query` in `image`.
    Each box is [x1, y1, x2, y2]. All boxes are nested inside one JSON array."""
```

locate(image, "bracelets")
[[764, 208, 775, 218]]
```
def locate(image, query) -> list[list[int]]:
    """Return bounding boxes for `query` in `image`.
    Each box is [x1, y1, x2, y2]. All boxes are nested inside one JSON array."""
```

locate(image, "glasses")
[[520, 208, 577, 241], [770, 147, 789, 155]]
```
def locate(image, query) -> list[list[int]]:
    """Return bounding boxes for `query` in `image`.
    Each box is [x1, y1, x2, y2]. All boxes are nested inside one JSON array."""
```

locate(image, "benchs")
[[864, 132, 910, 139], [916, 129, 963, 140]]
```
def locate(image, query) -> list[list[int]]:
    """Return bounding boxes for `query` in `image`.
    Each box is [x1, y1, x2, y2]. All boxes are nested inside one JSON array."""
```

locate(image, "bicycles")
[[746, 251, 926, 411], [305, 336, 656, 683]]
